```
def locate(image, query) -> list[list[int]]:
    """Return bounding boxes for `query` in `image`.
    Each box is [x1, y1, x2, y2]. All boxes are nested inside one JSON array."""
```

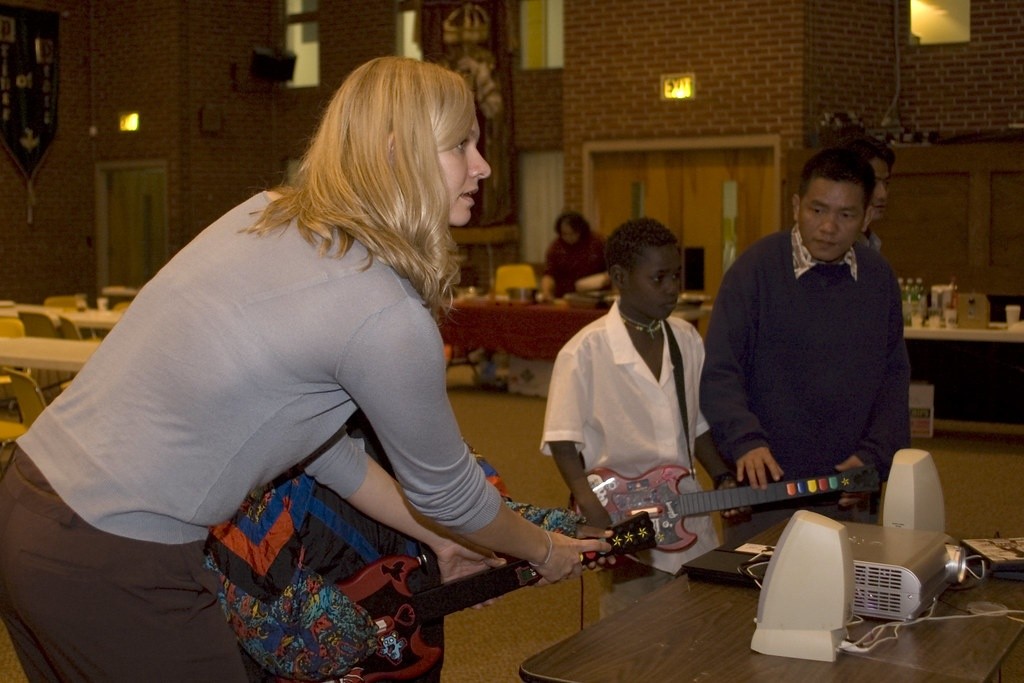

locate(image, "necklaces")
[[619, 309, 661, 340]]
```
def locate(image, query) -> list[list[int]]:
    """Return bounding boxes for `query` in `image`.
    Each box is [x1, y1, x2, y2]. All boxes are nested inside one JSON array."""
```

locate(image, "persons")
[[203, 408, 616, 681], [700, 136, 912, 554], [0, 57, 614, 683], [534, 209, 610, 303], [540, 217, 751, 575]]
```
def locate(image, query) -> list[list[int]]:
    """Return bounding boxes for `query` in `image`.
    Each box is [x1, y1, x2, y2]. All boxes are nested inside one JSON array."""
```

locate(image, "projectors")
[[838, 518, 968, 623]]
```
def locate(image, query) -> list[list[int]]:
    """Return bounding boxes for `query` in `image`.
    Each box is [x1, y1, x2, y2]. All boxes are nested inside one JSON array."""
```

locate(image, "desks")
[[898, 319, 1024, 343], [511, 514, 1024, 683], [437, 297, 712, 381], [0, 302, 130, 330], [0, 417, 32, 448], [0, 333, 107, 378]]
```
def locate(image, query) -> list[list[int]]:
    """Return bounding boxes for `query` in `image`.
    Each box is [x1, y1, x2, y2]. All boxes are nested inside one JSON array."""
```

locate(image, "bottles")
[[898, 278, 927, 325]]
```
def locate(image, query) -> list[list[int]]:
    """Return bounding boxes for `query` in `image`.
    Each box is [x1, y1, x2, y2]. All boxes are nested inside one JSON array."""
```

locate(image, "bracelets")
[[529, 530, 552, 566]]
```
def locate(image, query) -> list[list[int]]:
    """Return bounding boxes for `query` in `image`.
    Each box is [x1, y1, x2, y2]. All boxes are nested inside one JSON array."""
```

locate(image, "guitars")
[[568, 451, 889, 574], [313, 506, 660, 683]]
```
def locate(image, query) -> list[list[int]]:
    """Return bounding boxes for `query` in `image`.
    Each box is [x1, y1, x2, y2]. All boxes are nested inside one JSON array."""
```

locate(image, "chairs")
[[2, 364, 48, 477], [0, 317, 27, 338], [495, 263, 538, 300], [19, 309, 83, 340], [42, 296, 98, 339]]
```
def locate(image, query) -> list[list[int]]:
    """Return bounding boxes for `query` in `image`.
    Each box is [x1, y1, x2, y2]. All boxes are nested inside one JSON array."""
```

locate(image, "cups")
[[1005, 305, 1021, 327], [931, 284, 953, 309], [910, 309, 958, 329]]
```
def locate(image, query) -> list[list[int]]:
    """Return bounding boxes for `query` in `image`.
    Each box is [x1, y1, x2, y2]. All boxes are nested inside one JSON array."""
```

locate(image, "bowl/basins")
[[505, 287, 538, 302]]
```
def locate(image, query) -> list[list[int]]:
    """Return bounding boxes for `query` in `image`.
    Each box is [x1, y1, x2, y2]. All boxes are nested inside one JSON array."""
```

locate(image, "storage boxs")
[[955, 291, 990, 329], [506, 359, 553, 400]]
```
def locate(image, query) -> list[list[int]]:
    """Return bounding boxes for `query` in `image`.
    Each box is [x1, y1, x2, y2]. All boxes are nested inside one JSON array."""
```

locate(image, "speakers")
[[751, 509, 854, 662], [879, 450, 946, 534], [249, 49, 296, 83]]
